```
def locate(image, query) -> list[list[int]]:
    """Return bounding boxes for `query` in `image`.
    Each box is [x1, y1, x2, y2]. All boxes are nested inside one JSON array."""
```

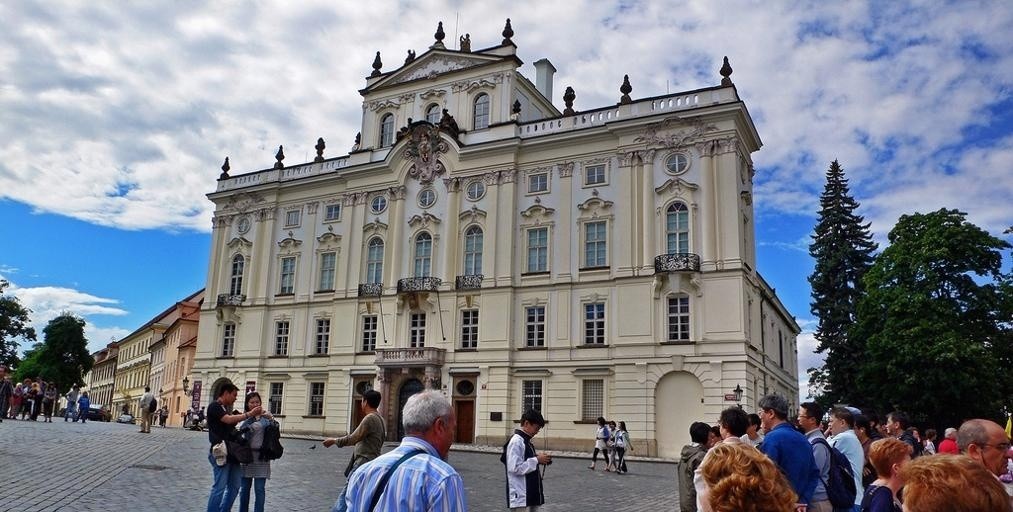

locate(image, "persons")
[[500, 409, 551, 512], [587, 417, 609, 471], [435, 108, 453, 128], [405, 49, 415, 65], [345, 390, 469, 512], [397, 118, 412, 140], [322, 390, 386, 512], [0, 365, 205, 433], [614, 421, 633, 473], [208, 383, 262, 512], [460, 34, 470, 52], [238, 391, 274, 512], [605, 421, 618, 472]]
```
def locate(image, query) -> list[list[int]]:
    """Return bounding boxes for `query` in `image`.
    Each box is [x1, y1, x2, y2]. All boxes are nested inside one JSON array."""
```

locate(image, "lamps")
[[732, 384, 743, 410], [157, 387, 166, 402], [365, 379, 371, 391], [180, 376, 194, 397]]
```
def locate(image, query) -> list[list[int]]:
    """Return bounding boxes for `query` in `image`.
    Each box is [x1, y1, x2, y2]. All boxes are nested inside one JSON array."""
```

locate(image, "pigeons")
[[309, 444, 316, 451]]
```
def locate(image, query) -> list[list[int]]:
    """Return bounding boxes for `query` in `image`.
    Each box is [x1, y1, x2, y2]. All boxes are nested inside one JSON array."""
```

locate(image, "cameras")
[[260, 409, 266, 415], [542, 454, 552, 465], [232, 410, 245, 422]]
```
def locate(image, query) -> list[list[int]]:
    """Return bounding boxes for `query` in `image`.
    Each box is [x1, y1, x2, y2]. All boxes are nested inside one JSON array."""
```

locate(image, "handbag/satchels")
[[150, 397, 157, 412], [230, 438, 253, 463], [261, 422, 283, 460], [42, 398, 48, 403], [812, 438, 857, 509], [607, 438, 615, 448]]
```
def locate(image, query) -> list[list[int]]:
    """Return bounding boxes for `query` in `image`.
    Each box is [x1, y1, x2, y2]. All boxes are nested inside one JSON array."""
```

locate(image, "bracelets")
[[245, 412, 248, 419]]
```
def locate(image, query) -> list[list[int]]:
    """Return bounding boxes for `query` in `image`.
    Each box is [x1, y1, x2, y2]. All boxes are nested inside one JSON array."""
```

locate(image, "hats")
[[212, 440, 228, 467]]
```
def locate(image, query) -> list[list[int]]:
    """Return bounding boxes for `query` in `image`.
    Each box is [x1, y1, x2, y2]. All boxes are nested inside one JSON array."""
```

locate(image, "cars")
[[116, 413, 135, 424], [86, 403, 111, 422]]
